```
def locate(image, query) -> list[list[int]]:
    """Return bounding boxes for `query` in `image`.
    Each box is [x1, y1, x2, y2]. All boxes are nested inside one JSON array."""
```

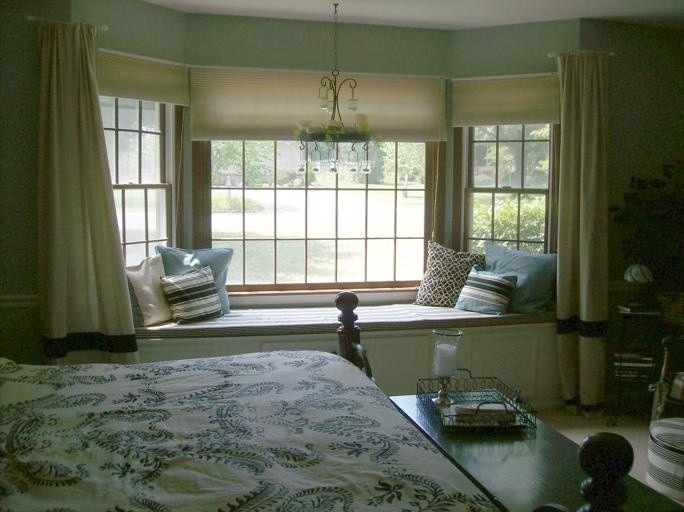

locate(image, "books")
[[451, 404, 516, 422], [617, 304, 661, 316], [612, 352, 656, 381]]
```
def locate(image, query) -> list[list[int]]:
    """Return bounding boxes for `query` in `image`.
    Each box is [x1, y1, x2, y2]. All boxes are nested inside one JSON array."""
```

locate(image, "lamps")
[[295, 3, 372, 174]]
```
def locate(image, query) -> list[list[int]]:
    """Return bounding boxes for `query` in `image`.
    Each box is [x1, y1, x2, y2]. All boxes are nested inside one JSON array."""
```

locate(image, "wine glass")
[[429, 327, 464, 405]]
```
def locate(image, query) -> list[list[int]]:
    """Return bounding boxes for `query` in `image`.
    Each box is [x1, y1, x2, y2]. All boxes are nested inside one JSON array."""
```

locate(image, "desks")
[[388, 390, 684, 512]]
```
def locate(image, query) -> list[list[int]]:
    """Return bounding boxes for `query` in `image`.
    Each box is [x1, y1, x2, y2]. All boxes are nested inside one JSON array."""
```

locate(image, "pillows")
[[125, 244, 234, 328], [412, 241, 557, 315]]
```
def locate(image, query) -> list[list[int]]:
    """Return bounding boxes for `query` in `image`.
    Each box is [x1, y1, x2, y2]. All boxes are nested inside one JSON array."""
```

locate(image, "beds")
[[0, 291, 502, 512]]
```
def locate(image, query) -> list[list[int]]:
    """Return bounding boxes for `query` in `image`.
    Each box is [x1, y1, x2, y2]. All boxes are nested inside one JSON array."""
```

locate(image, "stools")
[[646, 418, 684, 507]]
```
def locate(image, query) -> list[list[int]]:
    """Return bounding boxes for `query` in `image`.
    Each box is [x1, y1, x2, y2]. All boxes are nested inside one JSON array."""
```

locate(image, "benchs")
[[133, 301, 566, 413]]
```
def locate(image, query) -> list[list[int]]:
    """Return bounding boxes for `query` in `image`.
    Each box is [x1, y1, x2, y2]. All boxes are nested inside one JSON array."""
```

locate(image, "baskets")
[[417, 368, 537, 437]]
